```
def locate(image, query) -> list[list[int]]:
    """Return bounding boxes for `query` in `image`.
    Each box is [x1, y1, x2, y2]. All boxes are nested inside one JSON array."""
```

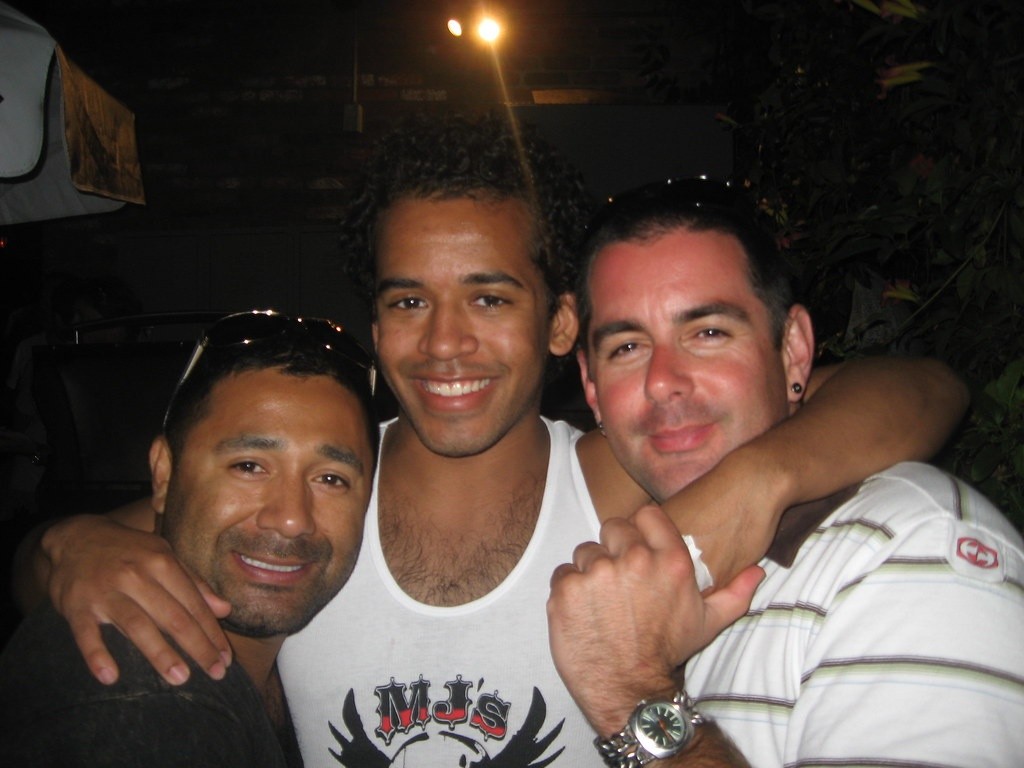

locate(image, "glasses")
[[161, 310, 377, 436], [582, 177, 774, 263]]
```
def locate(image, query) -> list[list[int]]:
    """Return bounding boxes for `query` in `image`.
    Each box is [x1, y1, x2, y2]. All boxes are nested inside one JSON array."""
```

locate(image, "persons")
[[11, 104, 971, 768], [551, 177, 1023, 767], [0, 310, 380, 768]]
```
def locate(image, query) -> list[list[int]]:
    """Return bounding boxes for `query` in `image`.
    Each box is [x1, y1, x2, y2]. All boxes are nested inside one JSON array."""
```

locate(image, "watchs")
[[593, 691, 704, 767]]
